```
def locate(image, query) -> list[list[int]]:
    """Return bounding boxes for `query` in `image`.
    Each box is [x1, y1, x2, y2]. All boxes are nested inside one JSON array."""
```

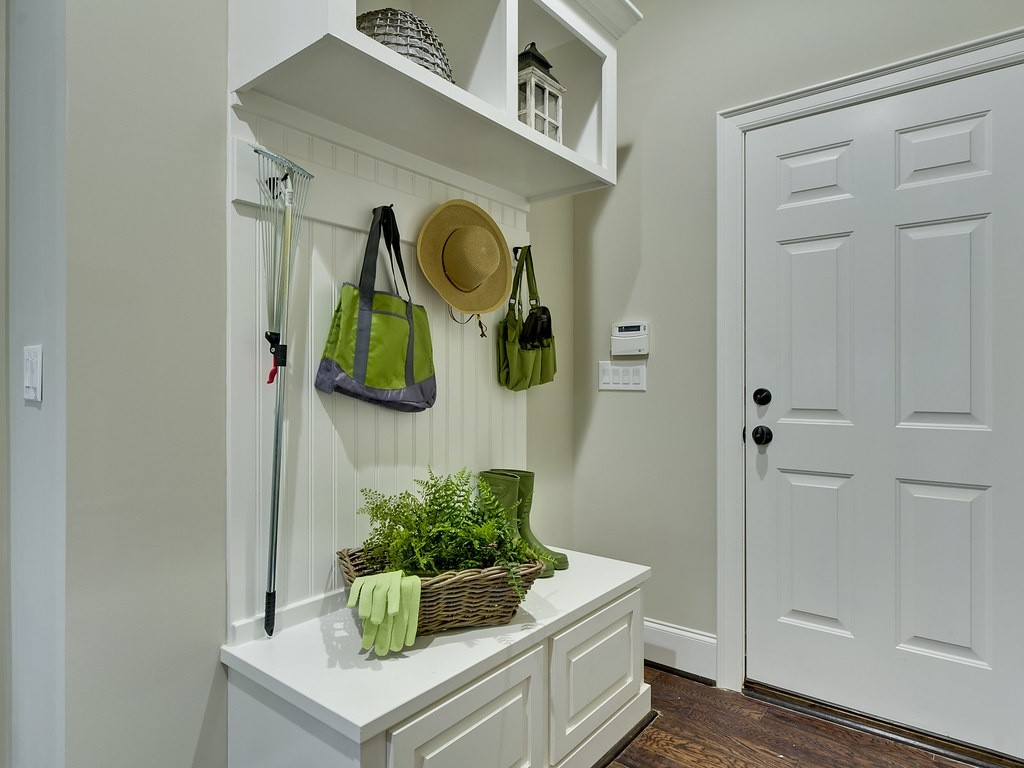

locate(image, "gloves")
[[347, 570, 421, 656]]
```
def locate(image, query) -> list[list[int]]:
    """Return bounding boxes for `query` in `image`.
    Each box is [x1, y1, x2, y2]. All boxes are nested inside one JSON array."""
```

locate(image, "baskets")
[[336, 544, 546, 634]]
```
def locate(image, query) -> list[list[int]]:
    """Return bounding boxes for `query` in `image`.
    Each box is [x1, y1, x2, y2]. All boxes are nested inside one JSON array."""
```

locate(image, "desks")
[[220, 543, 651, 768]]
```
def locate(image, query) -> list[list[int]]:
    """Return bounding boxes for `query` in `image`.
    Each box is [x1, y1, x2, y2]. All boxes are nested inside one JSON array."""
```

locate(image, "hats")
[[417, 199, 512, 315]]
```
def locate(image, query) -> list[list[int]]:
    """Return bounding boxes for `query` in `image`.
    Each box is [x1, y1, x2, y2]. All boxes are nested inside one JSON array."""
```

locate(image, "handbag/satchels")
[[496, 246, 558, 391], [314, 206, 436, 412]]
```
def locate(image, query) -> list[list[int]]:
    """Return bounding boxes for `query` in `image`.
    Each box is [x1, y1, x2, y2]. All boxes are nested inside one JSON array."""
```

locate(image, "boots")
[[477, 468, 569, 578]]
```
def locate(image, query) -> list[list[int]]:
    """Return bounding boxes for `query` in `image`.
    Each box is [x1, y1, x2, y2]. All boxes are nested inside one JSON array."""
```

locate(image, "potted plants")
[[335, 464, 545, 636]]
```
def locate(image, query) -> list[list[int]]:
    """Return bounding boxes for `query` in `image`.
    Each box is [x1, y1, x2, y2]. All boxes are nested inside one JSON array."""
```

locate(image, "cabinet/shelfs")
[[226, 1, 644, 212]]
